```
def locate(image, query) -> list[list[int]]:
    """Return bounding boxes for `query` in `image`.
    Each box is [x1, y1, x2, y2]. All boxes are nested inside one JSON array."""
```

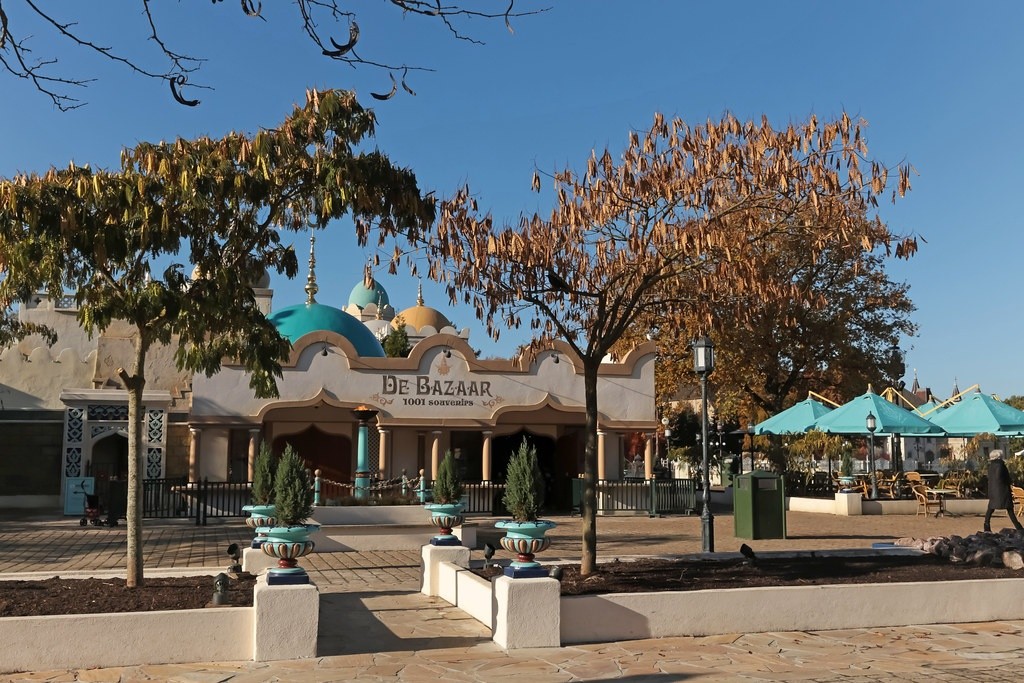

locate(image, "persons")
[[984, 450, 1023, 532]]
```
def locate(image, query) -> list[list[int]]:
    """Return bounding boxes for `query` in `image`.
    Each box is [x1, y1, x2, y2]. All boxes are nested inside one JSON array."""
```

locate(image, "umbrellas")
[[755, 384, 1024, 484]]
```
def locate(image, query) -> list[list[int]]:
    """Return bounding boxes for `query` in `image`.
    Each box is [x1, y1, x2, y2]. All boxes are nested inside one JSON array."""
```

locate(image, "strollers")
[[80, 480, 106, 527]]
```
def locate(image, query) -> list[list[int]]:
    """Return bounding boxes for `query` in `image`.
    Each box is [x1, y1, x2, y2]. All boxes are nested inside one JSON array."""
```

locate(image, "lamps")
[[321, 336, 328, 357], [443, 339, 452, 359], [551, 345, 560, 363]]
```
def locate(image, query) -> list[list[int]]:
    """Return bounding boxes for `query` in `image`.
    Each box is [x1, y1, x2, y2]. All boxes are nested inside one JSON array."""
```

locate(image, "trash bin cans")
[[733, 468, 786, 541], [569, 478, 584, 514], [106, 480, 128, 526], [625, 476, 646, 483], [720, 454, 743, 486]]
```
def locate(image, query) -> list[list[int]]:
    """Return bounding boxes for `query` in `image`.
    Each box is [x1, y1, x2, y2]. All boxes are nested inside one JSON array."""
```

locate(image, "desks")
[[919, 474, 938, 495], [852, 473, 869, 484], [925, 489, 958, 519]]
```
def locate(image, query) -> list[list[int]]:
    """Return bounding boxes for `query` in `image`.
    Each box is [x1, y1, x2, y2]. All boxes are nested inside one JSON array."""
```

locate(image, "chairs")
[[912, 485, 944, 518], [903, 472, 929, 499], [1011, 487, 1024, 515], [831, 469, 870, 500], [875, 471, 900, 499]]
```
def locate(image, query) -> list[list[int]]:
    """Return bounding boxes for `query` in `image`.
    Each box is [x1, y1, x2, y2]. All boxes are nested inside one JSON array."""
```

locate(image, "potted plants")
[[254, 443, 322, 586], [240, 439, 277, 549], [493, 435, 559, 579], [425, 447, 468, 547]]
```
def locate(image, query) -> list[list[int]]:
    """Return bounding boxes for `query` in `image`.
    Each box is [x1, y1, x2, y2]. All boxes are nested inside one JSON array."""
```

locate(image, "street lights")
[[866, 410, 881, 500], [662, 417, 672, 473], [748, 421, 756, 472], [717, 420, 724, 460], [692, 333, 717, 553]]
